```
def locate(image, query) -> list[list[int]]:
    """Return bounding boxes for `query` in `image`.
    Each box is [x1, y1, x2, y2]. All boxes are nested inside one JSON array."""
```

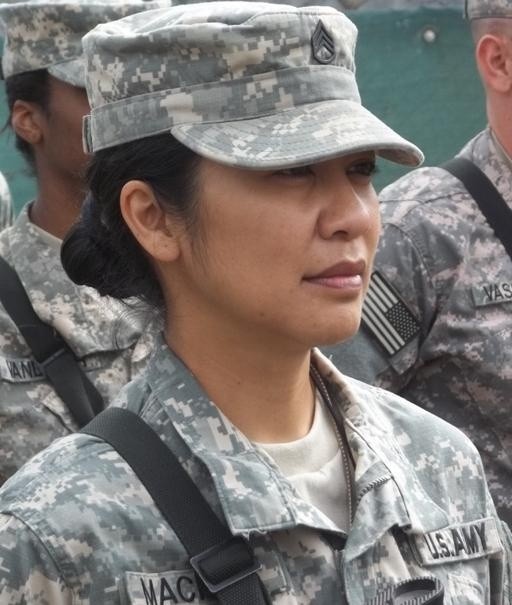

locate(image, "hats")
[[0, 0, 175, 91], [76, 0, 426, 170], [461, 0, 512, 18]]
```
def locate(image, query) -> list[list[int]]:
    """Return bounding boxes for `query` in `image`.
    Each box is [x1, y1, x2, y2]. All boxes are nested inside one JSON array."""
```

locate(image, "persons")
[[320, 1, 511, 530], [1, 2, 509, 605], [0, 2, 166, 478]]
